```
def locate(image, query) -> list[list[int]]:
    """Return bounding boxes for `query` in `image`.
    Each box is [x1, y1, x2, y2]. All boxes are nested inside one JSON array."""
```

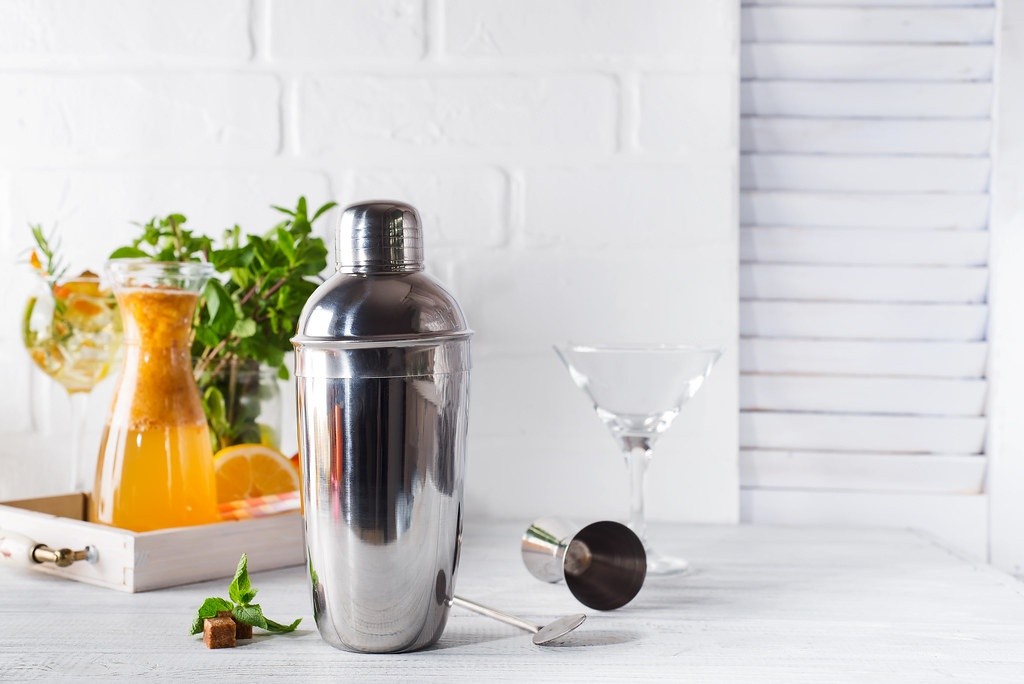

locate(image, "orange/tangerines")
[[209, 444, 299, 506]]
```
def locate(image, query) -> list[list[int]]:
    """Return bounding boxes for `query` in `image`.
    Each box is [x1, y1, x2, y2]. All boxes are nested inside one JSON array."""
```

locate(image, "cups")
[[290, 194, 473, 659], [521, 518, 649, 612]]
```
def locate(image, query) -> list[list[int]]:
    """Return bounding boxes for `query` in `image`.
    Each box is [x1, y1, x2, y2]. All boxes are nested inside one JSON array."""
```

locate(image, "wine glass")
[[557, 337, 725, 584]]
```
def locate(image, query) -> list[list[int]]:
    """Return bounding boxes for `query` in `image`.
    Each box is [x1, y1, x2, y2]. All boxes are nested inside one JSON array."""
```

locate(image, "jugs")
[[80, 258, 224, 536]]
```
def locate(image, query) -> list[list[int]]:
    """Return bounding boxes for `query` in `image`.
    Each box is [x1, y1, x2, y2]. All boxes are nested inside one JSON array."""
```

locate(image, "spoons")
[[452, 593, 587, 645]]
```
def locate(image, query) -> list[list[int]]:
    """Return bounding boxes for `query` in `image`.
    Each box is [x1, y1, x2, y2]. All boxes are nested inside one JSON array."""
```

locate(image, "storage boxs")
[[0, 493, 308, 593]]
